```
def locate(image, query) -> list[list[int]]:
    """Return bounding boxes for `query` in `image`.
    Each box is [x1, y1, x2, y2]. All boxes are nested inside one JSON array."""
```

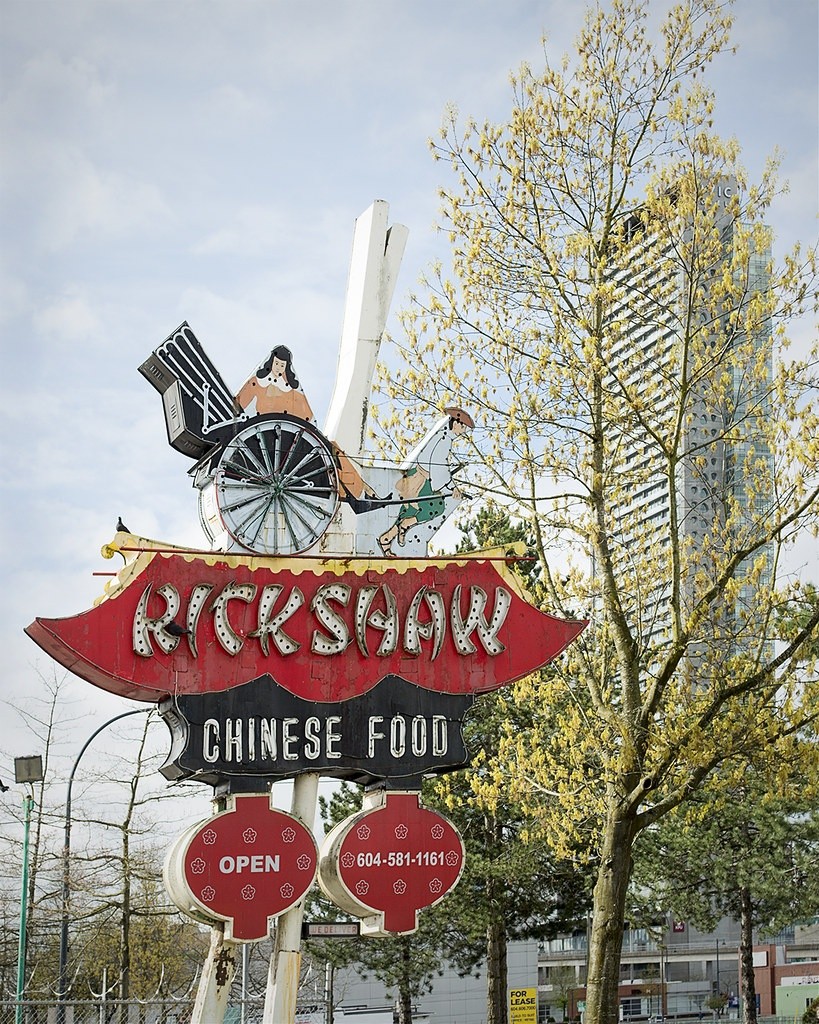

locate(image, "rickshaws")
[[138, 319, 454, 556]]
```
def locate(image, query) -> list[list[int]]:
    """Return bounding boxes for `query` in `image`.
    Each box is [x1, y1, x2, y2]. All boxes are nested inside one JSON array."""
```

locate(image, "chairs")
[[136, 320, 368, 512]]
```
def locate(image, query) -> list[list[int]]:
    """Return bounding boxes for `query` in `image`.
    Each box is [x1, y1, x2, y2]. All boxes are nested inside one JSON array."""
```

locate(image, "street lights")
[[717, 938, 726, 1019], [14, 754, 44, 1024]]
